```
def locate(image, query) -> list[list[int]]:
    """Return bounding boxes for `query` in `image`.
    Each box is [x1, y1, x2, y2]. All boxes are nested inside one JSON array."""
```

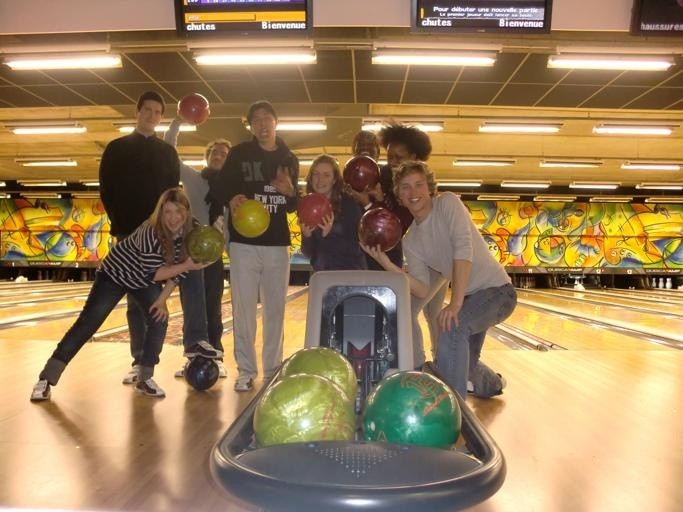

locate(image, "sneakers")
[[136, 379, 165, 397], [182, 340, 222, 359], [467, 373, 507, 395], [215, 360, 226, 377], [175, 365, 183, 377], [31, 379, 52, 400], [123, 365, 140, 384], [234, 375, 254, 391]]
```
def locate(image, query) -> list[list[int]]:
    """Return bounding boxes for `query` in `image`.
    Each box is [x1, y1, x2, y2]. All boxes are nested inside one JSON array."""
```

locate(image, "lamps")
[[115, 125, 196, 133], [5, 123, 87, 134], [453, 158, 514, 167], [2, 53, 123, 70], [1, 179, 102, 203], [13, 158, 76, 167], [362, 120, 444, 134], [369, 50, 498, 69], [592, 123, 673, 137], [295, 178, 309, 186], [478, 121, 562, 135], [620, 162, 683, 170], [539, 159, 602, 168], [181, 160, 208, 168], [243, 116, 328, 133], [295, 158, 339, 166], [192, 50, 317, 65], [377, 159, 388, 165], [547, 52, 678, 74], [435, 180, 682, 203]]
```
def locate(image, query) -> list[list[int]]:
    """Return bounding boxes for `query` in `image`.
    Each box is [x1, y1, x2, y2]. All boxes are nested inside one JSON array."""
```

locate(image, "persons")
[[154, 114, 231, 381], [349, 129, 381, 164], [209, 99, 301, 390], [293, 153, 370, 278], [26, 186, 217, 403], [96, 90, 184, 399], [357, 157, 519, 403], [342, 119, 433, 277]]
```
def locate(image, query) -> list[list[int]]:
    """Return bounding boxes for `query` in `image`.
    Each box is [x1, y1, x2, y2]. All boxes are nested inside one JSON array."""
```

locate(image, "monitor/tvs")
[[629, 1, 683, 36], [410, 0, 553, 34], [175, 0, 312, 40]]
